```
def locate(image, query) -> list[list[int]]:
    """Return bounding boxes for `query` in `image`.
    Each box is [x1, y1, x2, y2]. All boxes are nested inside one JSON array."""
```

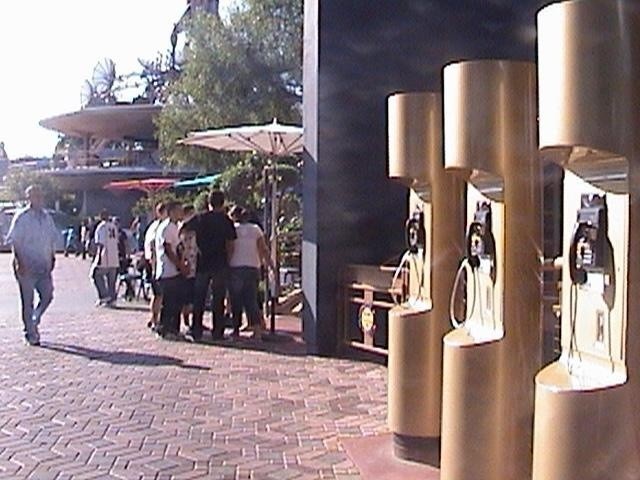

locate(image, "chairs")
[[114, 258, 148, 302]]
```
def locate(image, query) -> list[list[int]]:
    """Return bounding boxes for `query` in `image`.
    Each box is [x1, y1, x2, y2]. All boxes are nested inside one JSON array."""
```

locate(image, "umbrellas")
[[177, 174, 221, 185], [104, 177, 176, 219], [177, 117, 303, 332]]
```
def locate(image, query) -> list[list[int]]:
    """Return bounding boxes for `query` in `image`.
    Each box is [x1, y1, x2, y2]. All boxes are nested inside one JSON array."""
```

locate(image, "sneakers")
[[23, 325, 41, 346], [147, 318, 265, 344]]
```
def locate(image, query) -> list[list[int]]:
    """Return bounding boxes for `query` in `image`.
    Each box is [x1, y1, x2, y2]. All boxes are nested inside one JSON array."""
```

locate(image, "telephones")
[[404, 202, 425, 265], [466, 199, 492, 268], [569, 192, 607, 286]]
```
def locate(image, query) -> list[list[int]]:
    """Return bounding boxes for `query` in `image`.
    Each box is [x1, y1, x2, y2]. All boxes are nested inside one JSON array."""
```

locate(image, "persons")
[[61, 189, 271, 343], [4, 184, 59, 344]]
